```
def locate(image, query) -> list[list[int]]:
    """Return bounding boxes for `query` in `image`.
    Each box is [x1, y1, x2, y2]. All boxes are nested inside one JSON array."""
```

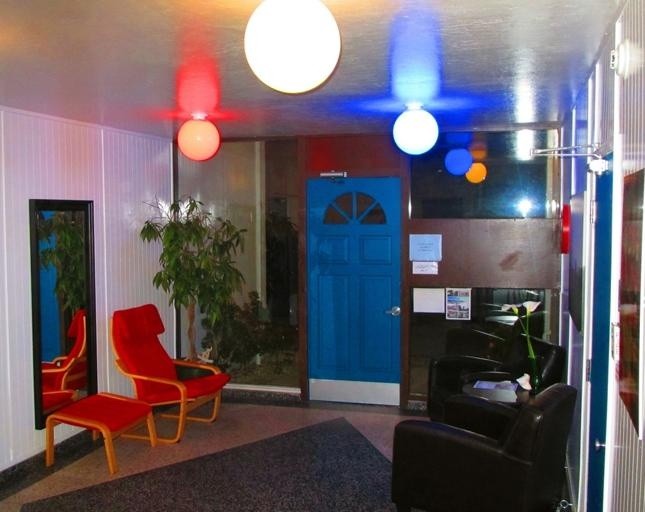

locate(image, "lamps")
[[176, 112, 222, 162], [392, 101, 440, 157]]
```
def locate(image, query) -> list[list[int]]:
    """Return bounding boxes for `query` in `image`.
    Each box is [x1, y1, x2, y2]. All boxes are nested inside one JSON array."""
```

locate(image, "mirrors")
[[409, 128, 554, 219], [28, 198, 98, 429]]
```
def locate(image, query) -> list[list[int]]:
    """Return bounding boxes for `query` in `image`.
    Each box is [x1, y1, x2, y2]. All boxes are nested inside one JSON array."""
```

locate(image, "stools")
[[47, 391, 156, 476]]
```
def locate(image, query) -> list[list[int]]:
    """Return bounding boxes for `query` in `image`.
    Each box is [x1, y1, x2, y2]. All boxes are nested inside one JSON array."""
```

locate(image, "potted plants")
[[138, 192, 248, 402]]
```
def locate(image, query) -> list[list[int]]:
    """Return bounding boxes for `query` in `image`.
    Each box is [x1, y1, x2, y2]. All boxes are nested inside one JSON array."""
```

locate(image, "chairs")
[[111, 302, 231, 445], [41, 308, 87, 414]]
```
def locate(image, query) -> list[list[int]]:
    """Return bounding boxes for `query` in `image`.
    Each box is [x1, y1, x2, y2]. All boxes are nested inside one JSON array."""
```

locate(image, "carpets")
[[19, 416, 392, 512]]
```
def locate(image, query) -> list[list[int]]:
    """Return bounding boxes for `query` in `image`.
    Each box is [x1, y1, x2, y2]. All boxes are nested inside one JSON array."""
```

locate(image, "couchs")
[[392, 331, 579, 512], [446, 287, 545, 343]]
